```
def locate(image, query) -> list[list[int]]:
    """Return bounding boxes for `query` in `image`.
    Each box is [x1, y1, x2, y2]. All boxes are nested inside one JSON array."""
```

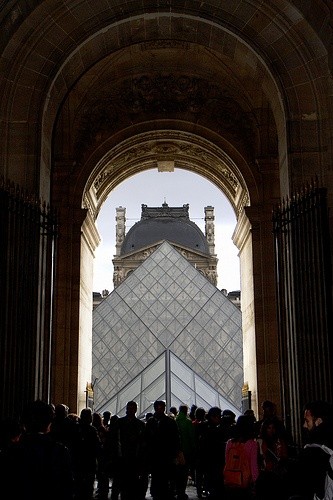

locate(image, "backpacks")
[[223, 440, 251, 488]]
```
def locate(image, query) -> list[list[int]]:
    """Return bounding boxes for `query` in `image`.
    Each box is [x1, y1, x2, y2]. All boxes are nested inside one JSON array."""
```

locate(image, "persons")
[[195, 407, 209, 499], [296, 400, 333, 500], [144, 399, 292, 486], [223, 415, 260, 500], [174, 405, 196, 499], [112, 400, 145, 500], [0, 399, 119, 500], [144, 400, 178, 500]]
